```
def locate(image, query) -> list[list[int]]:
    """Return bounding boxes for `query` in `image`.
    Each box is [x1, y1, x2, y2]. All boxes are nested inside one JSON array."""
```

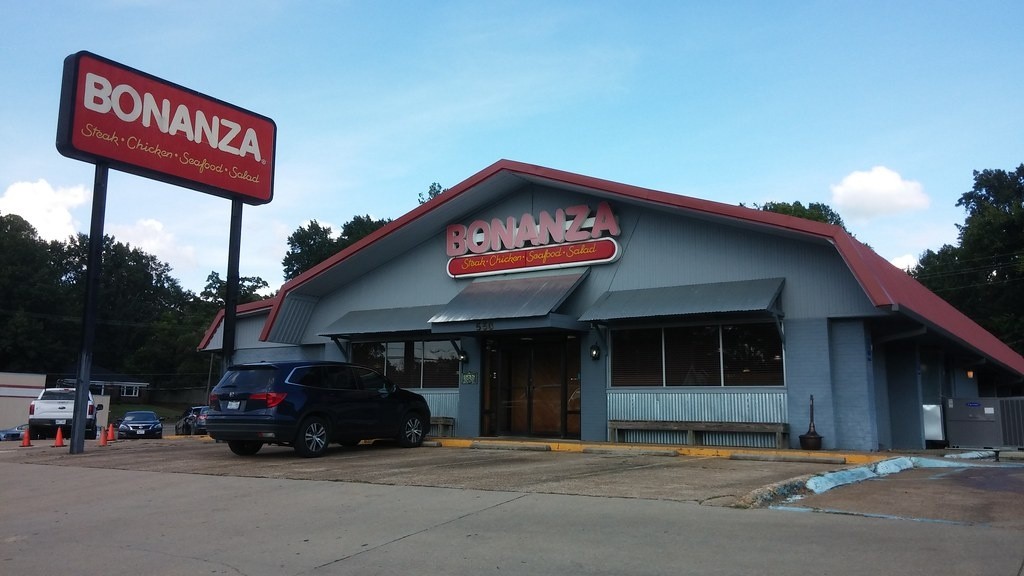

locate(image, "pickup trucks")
[[28, 387, 104, 439]]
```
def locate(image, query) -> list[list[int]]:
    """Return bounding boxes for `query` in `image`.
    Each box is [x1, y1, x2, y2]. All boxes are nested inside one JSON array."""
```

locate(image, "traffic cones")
[[107, 423, 117, 441], [96, 426, 111, 446], [19, 427, 34, 447], [50, 426, 68, 447]]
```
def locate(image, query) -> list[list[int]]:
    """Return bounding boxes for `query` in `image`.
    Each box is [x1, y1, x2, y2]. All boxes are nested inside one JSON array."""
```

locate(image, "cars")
[[174, 406, 202, 435], [0, 423, 29, 441], [117, 411, 165, 438], [194, 406, 213, 436]]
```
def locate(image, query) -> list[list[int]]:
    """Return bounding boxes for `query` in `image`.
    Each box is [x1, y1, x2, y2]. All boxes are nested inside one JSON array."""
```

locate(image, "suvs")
[[204, 360, 431, 458]]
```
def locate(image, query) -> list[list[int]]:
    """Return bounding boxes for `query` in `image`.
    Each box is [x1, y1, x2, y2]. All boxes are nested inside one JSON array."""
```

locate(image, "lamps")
[[458, 349, 469, 373], [590, 341, 601, 360], [967, 369, 974, 379]]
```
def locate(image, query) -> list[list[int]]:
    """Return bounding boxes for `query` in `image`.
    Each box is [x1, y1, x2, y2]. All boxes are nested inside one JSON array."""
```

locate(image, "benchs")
[[608, 420, 791, 449], [430, 416, 455, 438]]
